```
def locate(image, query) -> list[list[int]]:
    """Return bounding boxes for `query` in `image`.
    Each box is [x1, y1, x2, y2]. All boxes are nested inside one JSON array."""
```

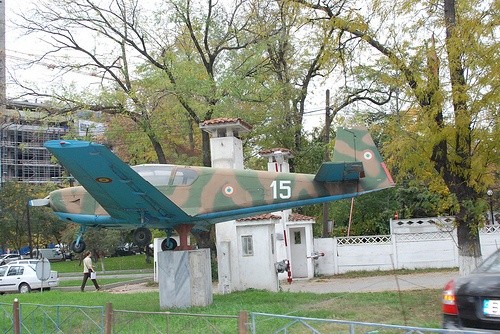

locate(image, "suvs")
[[109, 246, 136, 257], [0, 253, 24, 267]]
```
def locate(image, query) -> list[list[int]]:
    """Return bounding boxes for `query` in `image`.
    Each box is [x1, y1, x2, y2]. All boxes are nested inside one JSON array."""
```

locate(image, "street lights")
[[486, 187, 496, 231]]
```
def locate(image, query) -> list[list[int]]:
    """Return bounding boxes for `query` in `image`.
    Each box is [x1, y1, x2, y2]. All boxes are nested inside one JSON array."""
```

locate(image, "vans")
[[26, 248, 63, 263]]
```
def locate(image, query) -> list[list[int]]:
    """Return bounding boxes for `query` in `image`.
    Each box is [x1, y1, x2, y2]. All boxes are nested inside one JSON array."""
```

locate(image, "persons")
[[80, 250, 100, 293]]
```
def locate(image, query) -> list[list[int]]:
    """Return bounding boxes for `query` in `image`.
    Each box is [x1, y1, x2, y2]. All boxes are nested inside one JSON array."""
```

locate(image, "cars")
[[0, 265, 59, 297], [444, 247, 500, 334]]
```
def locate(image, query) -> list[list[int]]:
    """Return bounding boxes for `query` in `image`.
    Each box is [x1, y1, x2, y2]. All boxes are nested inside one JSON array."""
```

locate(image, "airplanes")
[[27, 124, 398, 254]]
